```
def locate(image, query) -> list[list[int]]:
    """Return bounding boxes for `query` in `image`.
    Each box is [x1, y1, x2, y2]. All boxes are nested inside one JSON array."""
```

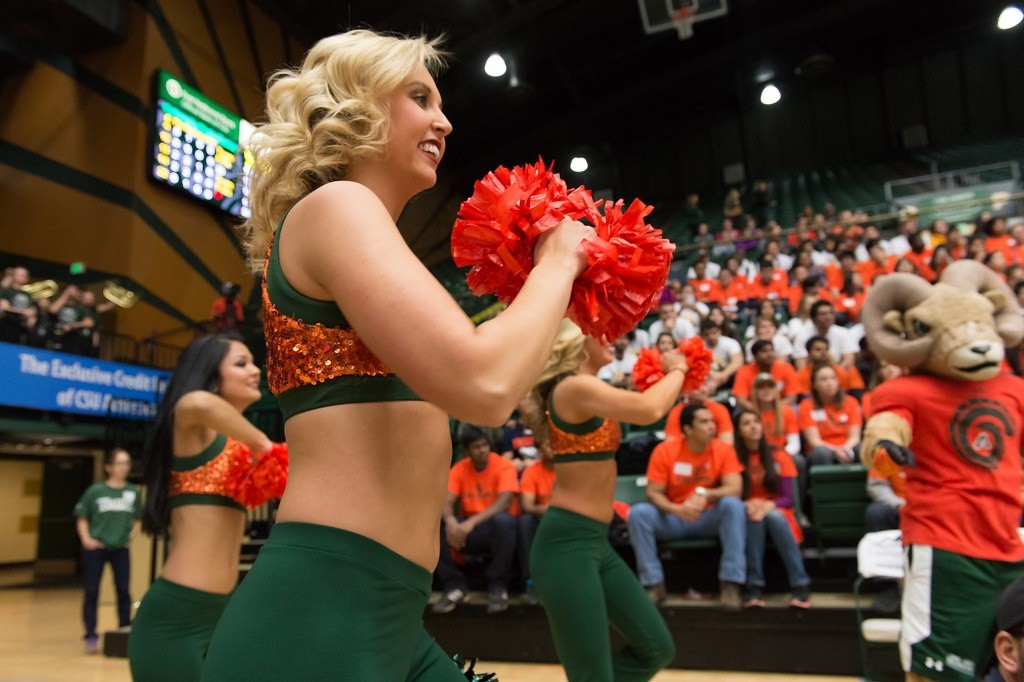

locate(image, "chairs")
[[613, 465, 872, 580]]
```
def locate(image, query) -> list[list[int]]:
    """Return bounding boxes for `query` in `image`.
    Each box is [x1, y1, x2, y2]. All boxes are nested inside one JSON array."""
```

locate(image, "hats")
[[755, 372, 773, 383], [996, 577, 1024, 630]]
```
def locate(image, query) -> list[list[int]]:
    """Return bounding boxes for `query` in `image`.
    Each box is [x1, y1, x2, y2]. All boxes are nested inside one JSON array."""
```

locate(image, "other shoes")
[[744, 599, 766, 607], [798, 514, 811, 528], [719, 581, 742, 610], [646, 582, 665, 603], [487, 586, 508, 613], [87, 639, 98, 654], [433, 587, 470, 612], [790, 598, 812, 609], [874, 588, 901, 616]]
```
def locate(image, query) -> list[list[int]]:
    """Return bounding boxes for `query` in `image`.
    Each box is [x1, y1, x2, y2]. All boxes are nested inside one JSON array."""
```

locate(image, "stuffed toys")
[[858, 259, 1024, 682]]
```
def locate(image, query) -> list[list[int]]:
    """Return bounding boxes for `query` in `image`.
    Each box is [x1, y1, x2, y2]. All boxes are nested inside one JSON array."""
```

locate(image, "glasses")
[[757, 383, 774, 388]]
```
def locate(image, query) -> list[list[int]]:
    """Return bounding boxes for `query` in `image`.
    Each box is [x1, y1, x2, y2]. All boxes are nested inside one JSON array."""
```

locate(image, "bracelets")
[[695, 486, 706, 496], [670, 367, 685, 376]]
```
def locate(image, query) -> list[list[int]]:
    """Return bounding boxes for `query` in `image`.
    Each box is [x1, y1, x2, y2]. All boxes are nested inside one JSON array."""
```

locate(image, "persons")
[[208, 283, 244, 330], [128, 335, 272, 682], [978, 576, 1024, 682], [201, 28, 601, 682], [597, 180, 1024, 607], [73, 447, 139, 656], [528, 318, 689, 682], [0, 267, 113, 358], [432, 410, 634, 614]]
